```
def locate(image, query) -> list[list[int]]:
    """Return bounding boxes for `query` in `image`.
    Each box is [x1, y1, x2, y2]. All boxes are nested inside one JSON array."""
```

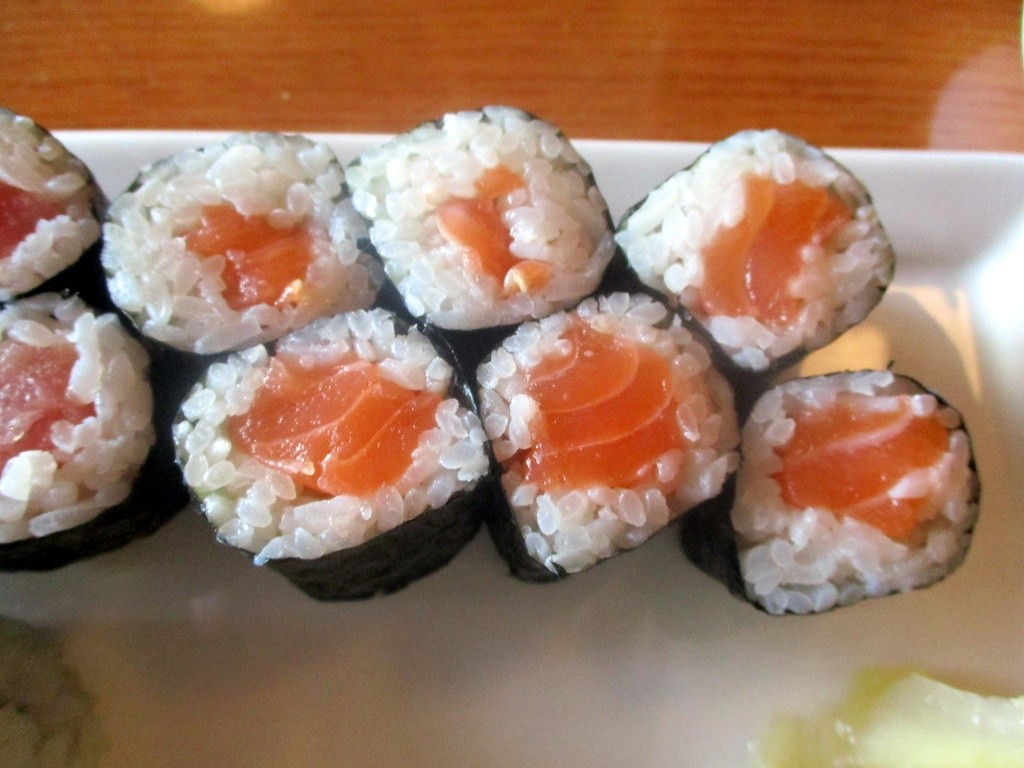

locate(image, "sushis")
[[0, 106, 983, 618]]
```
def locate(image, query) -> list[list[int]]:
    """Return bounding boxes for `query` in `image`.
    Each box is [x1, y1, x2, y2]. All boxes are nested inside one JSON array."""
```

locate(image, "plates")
[[0, 129, 1024, 768]]
